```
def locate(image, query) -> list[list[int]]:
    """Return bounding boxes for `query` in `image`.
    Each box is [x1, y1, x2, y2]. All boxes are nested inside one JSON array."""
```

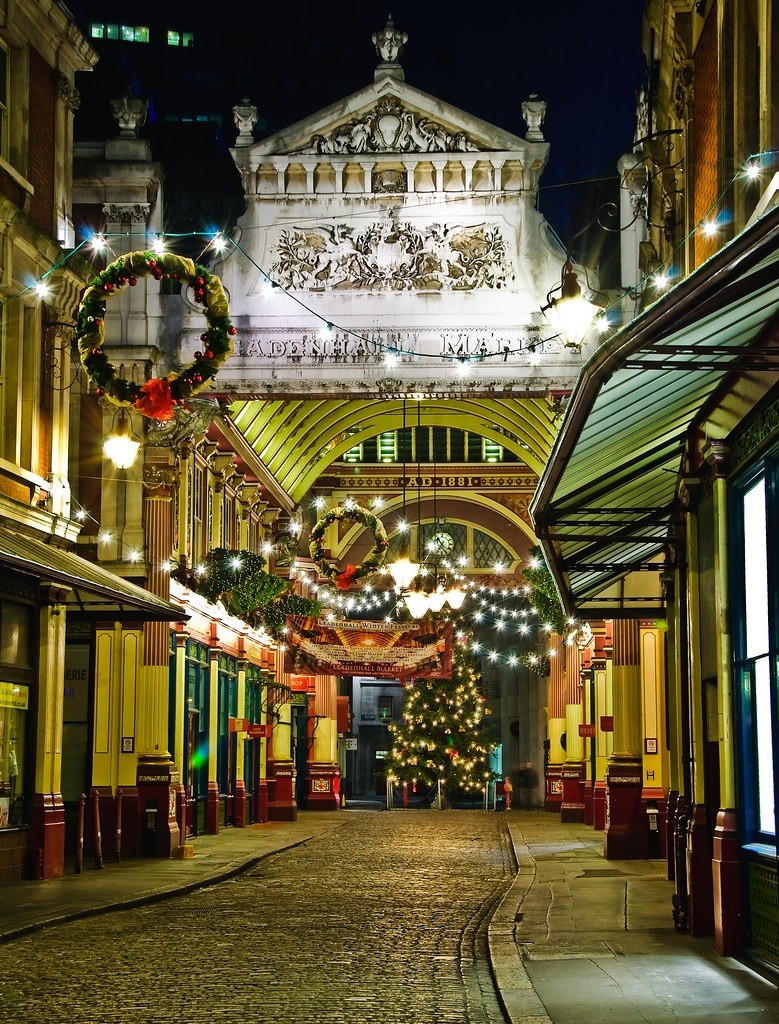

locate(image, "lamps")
[[390, 400, 465, 619], [101, 408, 142, 472], [543, 266, 639, 352]]
